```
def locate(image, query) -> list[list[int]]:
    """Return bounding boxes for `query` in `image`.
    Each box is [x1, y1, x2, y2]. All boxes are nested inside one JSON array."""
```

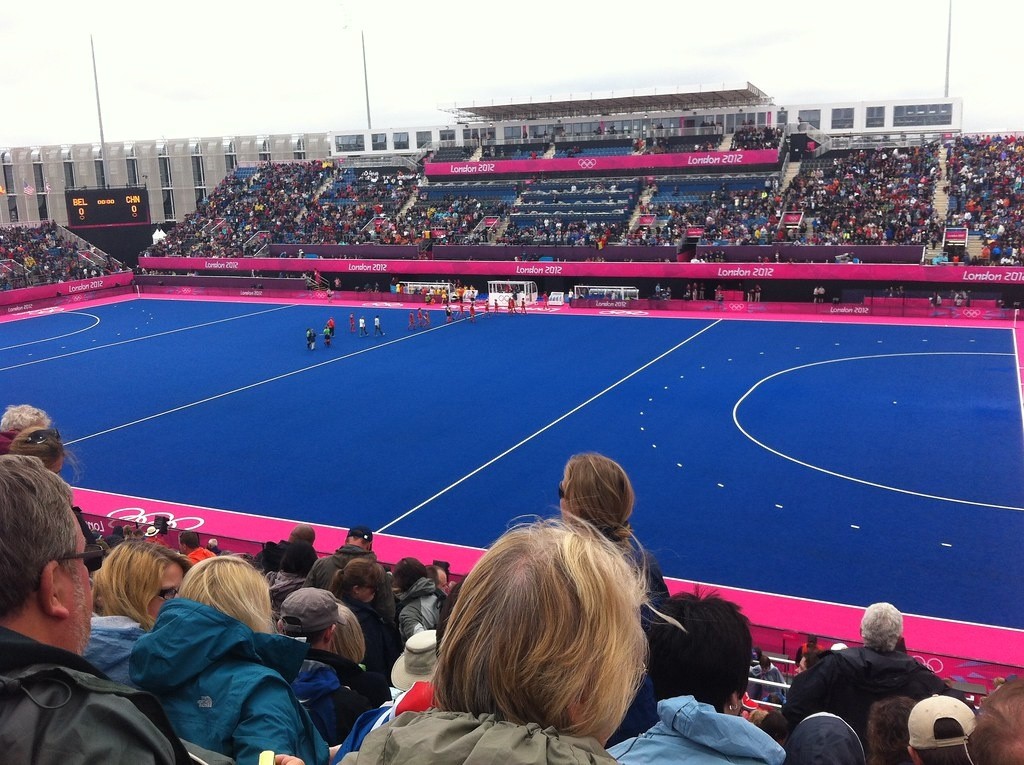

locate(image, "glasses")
[[24, 429, 61, 444], [38, 544, 106, 576], [558, 481, 569, 499], [761, 667, 768, 669], [367, 583, 379, 595], [158, 587, 178, 600]]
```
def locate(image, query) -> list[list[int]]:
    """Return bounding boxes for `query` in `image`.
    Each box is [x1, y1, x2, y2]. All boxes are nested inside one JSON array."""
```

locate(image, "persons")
[[0, 405, 1024, 765], [0, 117, 1024, 323], [310, 329, 316, 350], [323, 326, 331, 346], [374, 314, 385, 337], [423, 310, 431, 327], [469, 304, 476, 323], [559, 451, 671, 631], [335, 517, 648, 765], [542, 291, 549, 310], [128, 556, 342, 765], [349, 313, 356, 332], [782, 602, 973, 756], [417, 307, 424, 328], [327, 317, 335, 337], [358, 316, 369, 337], [493, 299, 499, 314], [568, 289, 574, 307], [520, 297, 528, 316], [408, 310, 416, 331], [482, 300, 491, 319], [457, 299, 467, 320]]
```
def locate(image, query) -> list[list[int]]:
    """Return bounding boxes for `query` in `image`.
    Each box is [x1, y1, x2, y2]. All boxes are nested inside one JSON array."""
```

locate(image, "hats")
[[907, 694, 977, 749], [347, 526, 373, 542], [741, 692, 758, 709], [280, 587, 347, 632], [831, 643, 849, 650], [145, 526, 160, 536], [390, 630, 436, 691]]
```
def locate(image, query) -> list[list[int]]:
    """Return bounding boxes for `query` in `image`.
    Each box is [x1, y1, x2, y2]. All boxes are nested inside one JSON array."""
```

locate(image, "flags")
[[22, 179, 34, 196], [0, 186, 7, 195], [44, 178, 52, 195]]
